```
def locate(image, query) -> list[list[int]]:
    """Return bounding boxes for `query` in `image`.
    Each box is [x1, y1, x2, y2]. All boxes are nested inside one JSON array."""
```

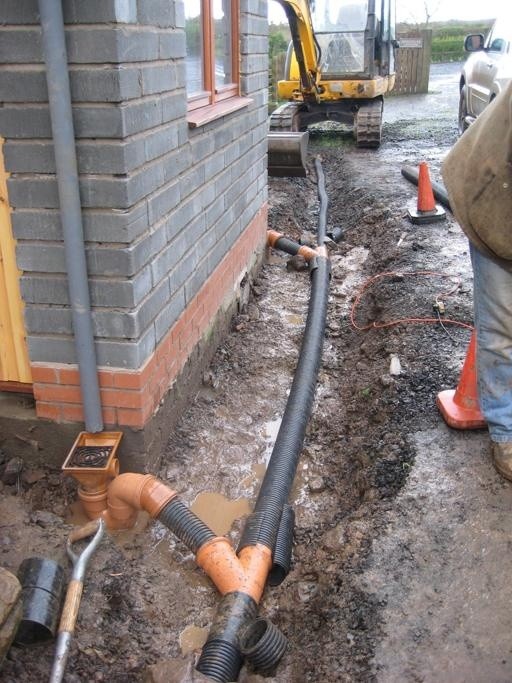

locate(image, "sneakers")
[[490, 441, 512, 480]]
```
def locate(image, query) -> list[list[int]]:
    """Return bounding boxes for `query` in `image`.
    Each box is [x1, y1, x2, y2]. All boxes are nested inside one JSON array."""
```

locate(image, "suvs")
[[456, 13, 512, 145]]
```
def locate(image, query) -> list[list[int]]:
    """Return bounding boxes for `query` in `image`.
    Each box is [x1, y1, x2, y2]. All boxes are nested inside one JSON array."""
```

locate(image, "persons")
[[437, 74, 512, 477]]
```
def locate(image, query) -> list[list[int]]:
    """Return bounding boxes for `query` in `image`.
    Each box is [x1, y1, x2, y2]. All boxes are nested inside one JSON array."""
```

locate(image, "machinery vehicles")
[[263, 0, 399, 176]]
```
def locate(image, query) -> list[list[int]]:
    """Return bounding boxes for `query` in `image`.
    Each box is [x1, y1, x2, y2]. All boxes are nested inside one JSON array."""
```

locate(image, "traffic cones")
[[432, 328, 488, 433], [405, 161, 448, 225]]
